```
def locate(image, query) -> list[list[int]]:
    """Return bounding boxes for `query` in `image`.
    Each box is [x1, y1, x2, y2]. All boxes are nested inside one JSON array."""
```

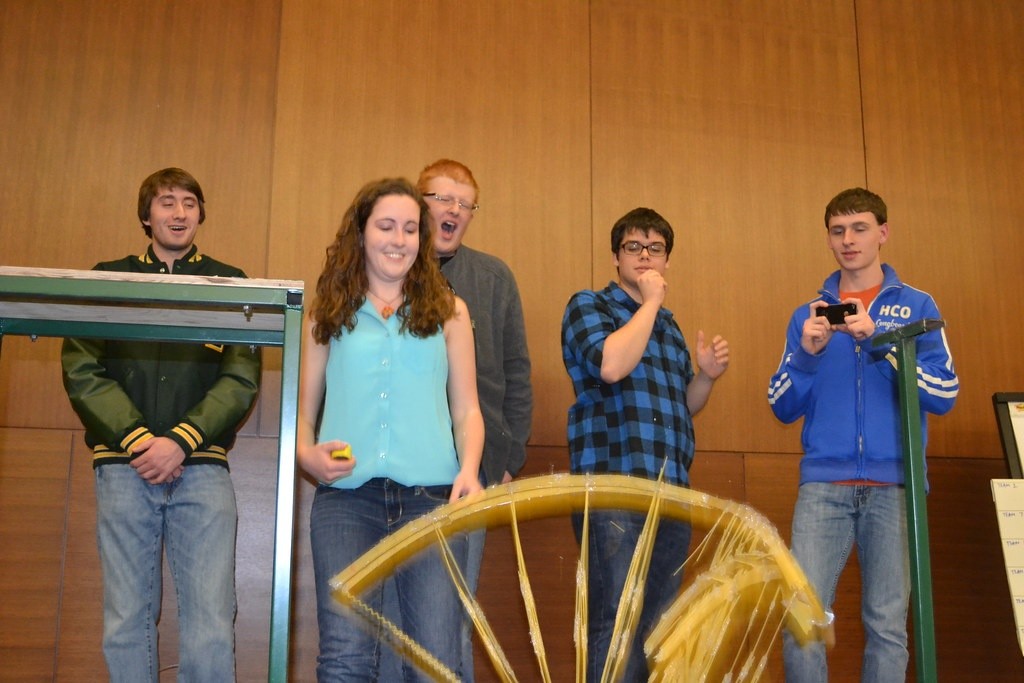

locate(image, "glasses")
[[423, 192, 479, 212], [619, 240, 668, 257]]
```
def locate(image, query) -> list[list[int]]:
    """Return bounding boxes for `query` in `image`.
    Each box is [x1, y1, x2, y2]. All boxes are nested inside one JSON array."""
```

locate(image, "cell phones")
[[816, 302, 856, 324]]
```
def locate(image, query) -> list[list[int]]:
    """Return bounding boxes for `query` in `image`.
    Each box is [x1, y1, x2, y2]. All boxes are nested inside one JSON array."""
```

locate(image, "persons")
[[559, 206, 731, 682], [417, 160, 533, 683], [60, 169, 265, 683], [766, 188, 962, 683], [295, 177, 486, 683]]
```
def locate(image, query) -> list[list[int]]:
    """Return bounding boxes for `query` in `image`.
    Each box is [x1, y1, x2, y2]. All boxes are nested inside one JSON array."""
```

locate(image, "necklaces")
[[368, 286, 404, 320]]
